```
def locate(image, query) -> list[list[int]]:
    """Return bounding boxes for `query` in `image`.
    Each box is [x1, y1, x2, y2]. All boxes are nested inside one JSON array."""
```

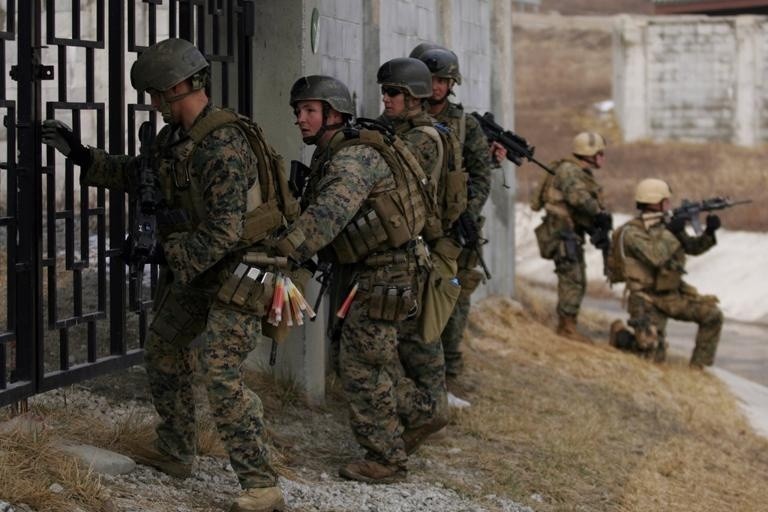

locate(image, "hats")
[[571, 133, 606, 158], [635, 179, 672, 205]]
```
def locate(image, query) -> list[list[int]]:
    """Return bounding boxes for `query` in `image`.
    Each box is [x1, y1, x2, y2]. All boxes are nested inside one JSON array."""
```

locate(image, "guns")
[[269, 159, 312, 366], [452, 213, 491, 280], [125, 121, 158, 348], [470, 111, 556, 176], [641, 196, 753, 237], [310, 243, 338, 323], [591, 185, 611, 275]]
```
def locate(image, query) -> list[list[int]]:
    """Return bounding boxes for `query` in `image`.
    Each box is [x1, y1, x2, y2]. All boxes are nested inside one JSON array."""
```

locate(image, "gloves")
[[667, 218, 685, 236], [41, 121, 85, 167], [589, 214, 611, 248], [707, 214, 721, 233]]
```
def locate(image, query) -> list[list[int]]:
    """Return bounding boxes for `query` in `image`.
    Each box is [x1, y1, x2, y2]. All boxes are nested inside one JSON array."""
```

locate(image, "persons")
[[409, 43, 492, 383], [531, 132, 613, 346], [40, 37, 289, 511], [371, 58, 463, 411], [489, 141, 506, 163], [609, 178, 724, 379], [278, 75, 447, 483]]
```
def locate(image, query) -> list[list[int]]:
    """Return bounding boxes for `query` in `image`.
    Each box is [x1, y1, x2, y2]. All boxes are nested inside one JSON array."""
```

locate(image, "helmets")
[[291, 74, 353, 115], [131, 38, 208, 90], [375, 39, 462, 99]]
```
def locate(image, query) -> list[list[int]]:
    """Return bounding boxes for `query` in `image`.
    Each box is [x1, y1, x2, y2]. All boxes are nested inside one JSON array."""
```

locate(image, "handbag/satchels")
[[422, 260, 462, 346]]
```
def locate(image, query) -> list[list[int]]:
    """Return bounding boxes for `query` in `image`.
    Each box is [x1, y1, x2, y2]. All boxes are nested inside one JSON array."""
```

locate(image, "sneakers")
[[340, 373, 476, 483], [120, 438, 191, 480], [232, 487, 283, 512], [610, 317, 625, 346], [557, 316, 594, 344]]
